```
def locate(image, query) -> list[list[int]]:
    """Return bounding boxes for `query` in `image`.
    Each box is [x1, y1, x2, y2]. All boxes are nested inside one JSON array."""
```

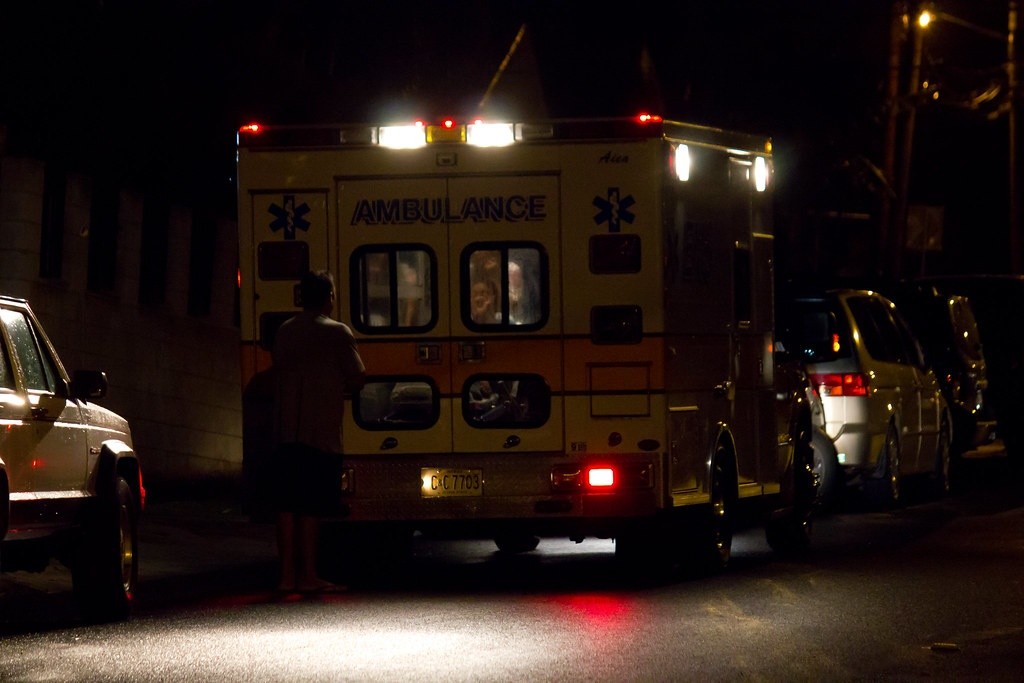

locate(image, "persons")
[[271, 272, 369, 595], [362, 246, 542, 329]]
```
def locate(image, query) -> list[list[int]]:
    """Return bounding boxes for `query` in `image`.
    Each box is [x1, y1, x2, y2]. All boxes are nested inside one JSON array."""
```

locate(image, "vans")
[[235, 112, 816, 586]]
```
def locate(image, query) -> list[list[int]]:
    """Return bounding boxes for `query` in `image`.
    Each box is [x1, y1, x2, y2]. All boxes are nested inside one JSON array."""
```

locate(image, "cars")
[[0, 292, 149, 615], [775, 284, 953, 509], [891, 285, 988, 441]]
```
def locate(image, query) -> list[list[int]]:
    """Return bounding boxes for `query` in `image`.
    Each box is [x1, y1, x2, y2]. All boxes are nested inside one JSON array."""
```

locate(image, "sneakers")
[[277, 575, 348, 605]]
